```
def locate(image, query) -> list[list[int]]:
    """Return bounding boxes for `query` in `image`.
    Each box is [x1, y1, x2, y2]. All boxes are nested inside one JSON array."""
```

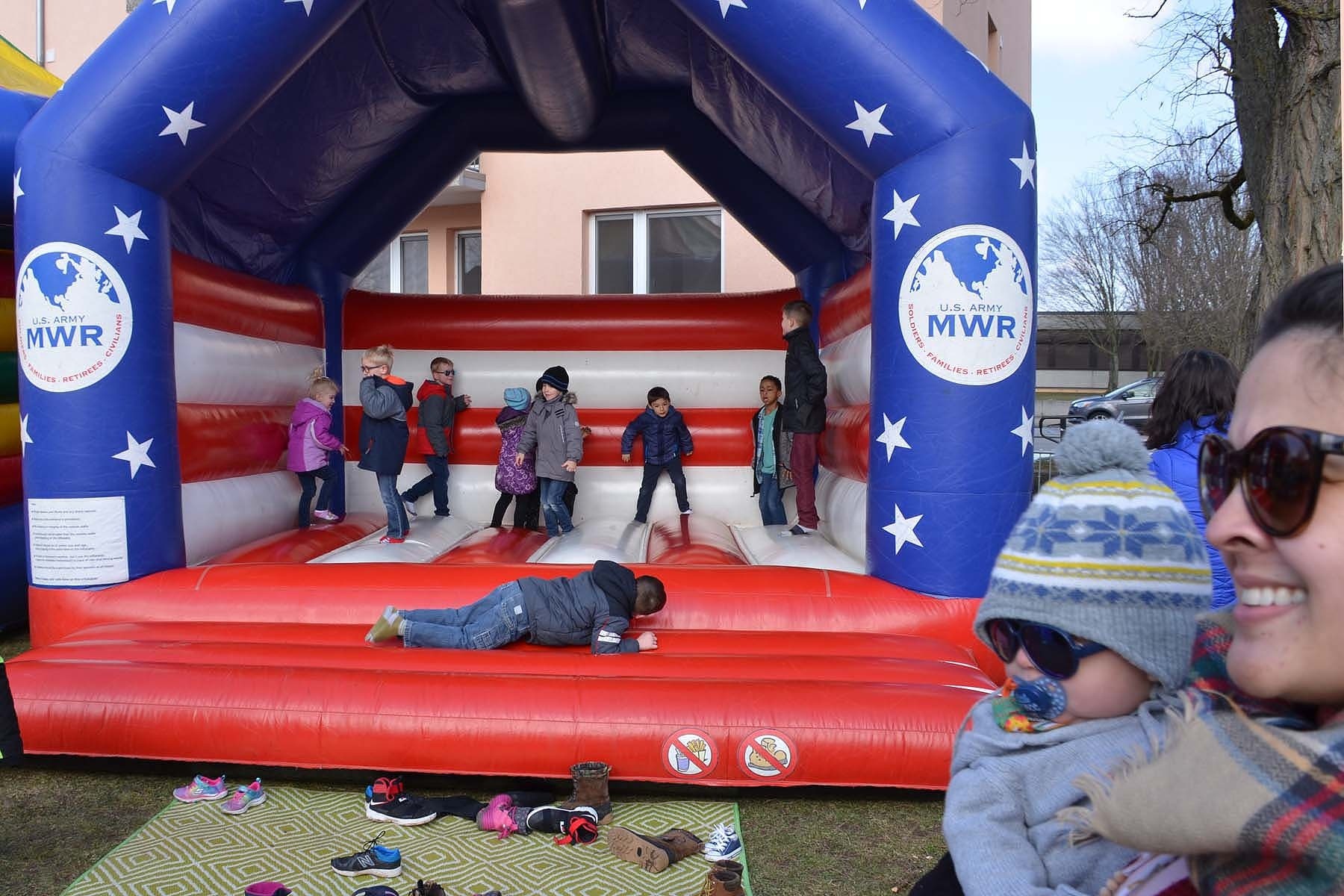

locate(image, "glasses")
[[362, 365, 384, 372], [1195, 425, 1344, 538], [988, 619, 1103, 680], [437, 371, 455, 376]]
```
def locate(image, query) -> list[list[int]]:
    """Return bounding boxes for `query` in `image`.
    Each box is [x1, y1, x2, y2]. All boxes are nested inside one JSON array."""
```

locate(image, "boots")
[[609, 827, 704, 873], [698, 860, 743, 896], [560, 761, 615, 826], [475, 795, 534, 837]]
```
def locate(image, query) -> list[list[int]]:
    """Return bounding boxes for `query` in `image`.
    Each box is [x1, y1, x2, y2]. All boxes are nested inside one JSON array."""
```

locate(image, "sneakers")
[[364, 778, 437, 825], [331, 831, 402, 878], [172, 774, 228, 802], [704, 834, 741, 862], [700, 825, 736, 853], [527, 806, 599, 845], [221, 777, 267, 813]]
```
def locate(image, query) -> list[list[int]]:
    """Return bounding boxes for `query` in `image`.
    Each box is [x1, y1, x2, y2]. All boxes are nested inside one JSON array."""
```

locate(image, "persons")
[[750, 375, 796, 526], [526, 376, 592, 531], [781, 300, 828, 537], [489, 388, 538, 527], [365, 560, 667, 653], [909, 261, 1344, 896], [1143, 350, 1240, 610], [287, 363, 349, 527], [516, 366, 583, 536], [943, 409, 1213, 896], [621, 387, 693, 523], [401, 357, 472, 519], [358, 343, 414, 544]]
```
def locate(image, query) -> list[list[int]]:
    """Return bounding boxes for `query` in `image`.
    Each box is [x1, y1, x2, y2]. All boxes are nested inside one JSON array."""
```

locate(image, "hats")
[[504, 388, 530, 410], [542, 366, 569, 393], [971, 423, 1212, 694]]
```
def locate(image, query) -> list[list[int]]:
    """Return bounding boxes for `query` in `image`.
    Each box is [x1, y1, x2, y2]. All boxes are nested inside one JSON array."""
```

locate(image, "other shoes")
[[245, 881, 292, 896], [409, 879, 446, 896], [354, 886, 399, 896]]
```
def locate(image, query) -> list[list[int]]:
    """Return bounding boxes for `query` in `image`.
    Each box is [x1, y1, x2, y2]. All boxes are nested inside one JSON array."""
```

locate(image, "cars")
[[1068, 375, 1166, 434]]
